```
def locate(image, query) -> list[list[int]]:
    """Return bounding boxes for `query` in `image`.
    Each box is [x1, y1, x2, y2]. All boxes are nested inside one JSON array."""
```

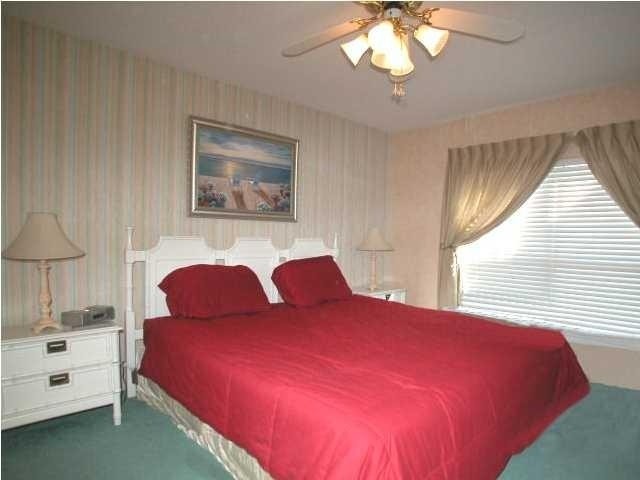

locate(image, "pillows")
[[156, 263, 272, 319], [272, 254, 354, 307]]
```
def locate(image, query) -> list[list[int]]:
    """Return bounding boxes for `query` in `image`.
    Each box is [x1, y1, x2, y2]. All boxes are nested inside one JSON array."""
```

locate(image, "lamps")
[[355, 229, 395, 292], [339, 19, 450, 101], [2, 210, 88, 334]]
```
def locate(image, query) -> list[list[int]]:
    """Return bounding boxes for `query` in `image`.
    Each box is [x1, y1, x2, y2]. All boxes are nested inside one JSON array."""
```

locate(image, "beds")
[[122, 225, 591, 479]]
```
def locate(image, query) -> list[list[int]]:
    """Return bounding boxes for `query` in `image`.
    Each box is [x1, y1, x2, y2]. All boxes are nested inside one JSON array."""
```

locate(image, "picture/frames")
[[188, 116, 301, 223]]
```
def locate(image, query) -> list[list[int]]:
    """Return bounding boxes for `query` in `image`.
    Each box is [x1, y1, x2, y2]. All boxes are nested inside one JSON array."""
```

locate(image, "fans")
[[282, 1, 528, 56]]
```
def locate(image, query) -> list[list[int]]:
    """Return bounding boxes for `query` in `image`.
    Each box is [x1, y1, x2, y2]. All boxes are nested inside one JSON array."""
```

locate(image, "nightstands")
[[1, 322, 121, 435], [356, 283, 406, 304]]
